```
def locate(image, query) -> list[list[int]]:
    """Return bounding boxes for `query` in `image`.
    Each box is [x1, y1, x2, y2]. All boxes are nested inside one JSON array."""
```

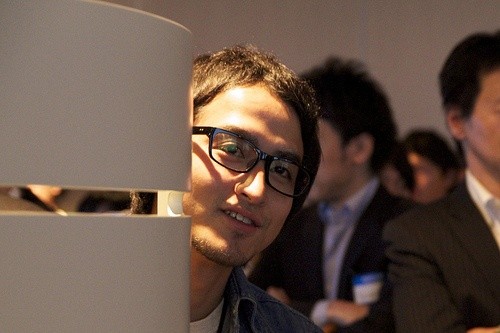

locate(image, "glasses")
[[191, 124, 310, 198]]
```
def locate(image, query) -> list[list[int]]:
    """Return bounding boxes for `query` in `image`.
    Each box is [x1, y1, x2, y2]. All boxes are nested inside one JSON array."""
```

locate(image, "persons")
[[114, 43, 326, 332], [394, 126, 459, 205], [384, 29, 500, 333], [246, 54, 416, 332]]
